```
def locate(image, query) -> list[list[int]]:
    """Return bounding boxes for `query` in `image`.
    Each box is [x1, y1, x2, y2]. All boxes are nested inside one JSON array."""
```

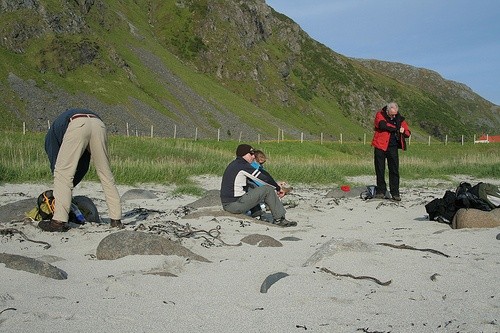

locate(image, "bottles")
[[70, 203, 86, 222]]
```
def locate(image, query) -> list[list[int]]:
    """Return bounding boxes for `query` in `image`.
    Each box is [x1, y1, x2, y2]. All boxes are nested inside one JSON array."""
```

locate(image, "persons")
[[250, 150, 285, 211], [371, 104, 411, 201], [221, 144, 297, 227], [38, 108, 125, 231]]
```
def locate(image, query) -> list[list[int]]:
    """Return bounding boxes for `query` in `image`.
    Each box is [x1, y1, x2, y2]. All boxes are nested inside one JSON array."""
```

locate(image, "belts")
[[72, 115, 97, 120]]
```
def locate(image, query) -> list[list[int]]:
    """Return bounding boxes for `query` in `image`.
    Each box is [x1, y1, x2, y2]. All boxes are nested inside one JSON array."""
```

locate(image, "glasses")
[[390, 114, 397, 116], [248, 151, 254, 156]]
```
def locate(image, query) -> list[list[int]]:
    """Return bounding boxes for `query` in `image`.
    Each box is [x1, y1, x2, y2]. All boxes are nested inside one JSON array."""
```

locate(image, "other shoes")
[[110, 219, 125, 229], [272, 218, 297, 226], [38, 220, 69, 232], [375, 193, 401, 201]]
